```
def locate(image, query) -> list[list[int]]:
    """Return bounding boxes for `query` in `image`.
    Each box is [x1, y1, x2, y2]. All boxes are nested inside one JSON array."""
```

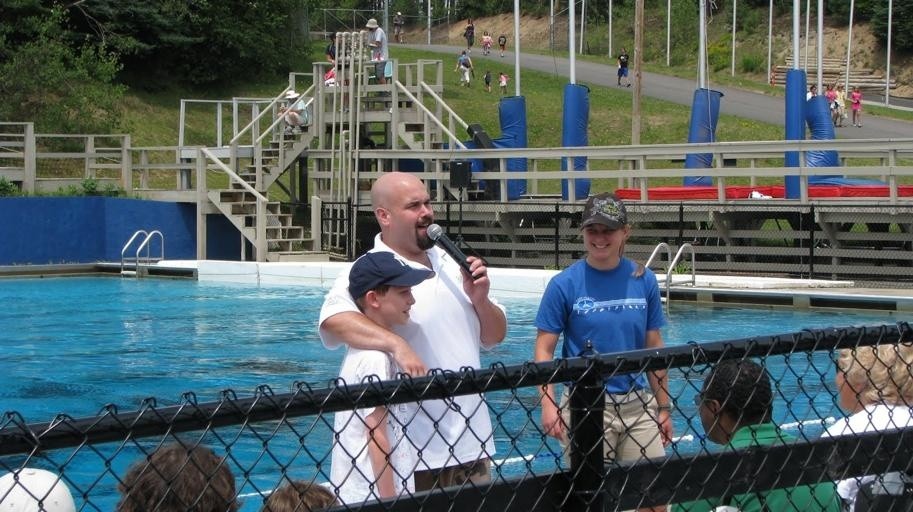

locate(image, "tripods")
[[454, 187, 489, 267]]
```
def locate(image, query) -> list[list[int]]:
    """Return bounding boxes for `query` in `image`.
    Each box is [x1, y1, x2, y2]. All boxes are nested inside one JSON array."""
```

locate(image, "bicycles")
[[399, 29, 409, 49]]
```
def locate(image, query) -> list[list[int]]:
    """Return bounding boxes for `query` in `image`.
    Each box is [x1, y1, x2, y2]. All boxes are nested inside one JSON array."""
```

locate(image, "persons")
[[318, 172, 509, 487], [820, 342, 913, 509], [324, 10, 404, 95], [114, 440, 238, 511], [534, 192, 673, 512], [331, 253, 433, 505], [278, 90, 309, 134], [807, 81, 862, 129], [455, 18, 508, 97], [616, 46, 631, 89], [674, 360, 840, 512], [261, 485, 340, 512]]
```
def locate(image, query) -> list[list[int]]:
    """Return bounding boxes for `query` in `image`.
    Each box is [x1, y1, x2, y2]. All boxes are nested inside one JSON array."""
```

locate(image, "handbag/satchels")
[[462, 58, 470, 67]]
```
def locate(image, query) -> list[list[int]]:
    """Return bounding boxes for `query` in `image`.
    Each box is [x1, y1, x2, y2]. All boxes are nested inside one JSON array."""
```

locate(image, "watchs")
[[659, 402, 674, 414]]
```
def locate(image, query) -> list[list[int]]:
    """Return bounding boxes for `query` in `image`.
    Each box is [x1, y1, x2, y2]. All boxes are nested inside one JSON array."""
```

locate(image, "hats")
[[366, 19, 379, 29], [285, 90, 300, 99], [397, 12, 401, 15], [349, 251, 436, 299], [580, 190, 627, 232]]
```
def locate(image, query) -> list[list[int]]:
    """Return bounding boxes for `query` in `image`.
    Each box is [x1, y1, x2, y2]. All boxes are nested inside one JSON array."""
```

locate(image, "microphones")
[[427, 224, 482, 281]]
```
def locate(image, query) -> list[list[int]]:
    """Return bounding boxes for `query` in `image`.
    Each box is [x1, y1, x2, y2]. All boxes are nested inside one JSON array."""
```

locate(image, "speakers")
[[450, 162, 471, 187]]
